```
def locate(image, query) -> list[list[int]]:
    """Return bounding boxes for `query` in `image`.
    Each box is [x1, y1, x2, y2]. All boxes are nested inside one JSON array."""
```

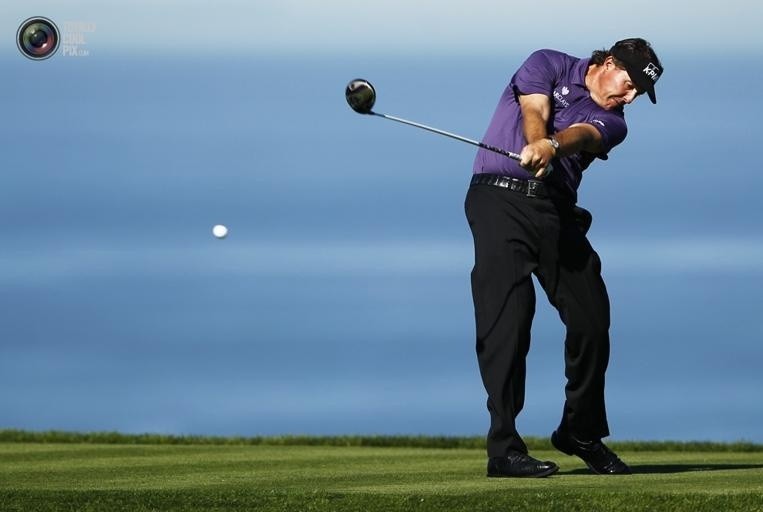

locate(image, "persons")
[[463, 36, 666, 478]]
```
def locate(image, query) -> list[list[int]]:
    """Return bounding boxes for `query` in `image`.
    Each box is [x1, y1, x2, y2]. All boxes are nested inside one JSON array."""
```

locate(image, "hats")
[[611, 45, 663, 104]]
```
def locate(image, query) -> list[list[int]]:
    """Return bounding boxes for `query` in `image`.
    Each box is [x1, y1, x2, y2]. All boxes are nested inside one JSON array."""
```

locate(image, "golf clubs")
[[346, 80, 520, 161]]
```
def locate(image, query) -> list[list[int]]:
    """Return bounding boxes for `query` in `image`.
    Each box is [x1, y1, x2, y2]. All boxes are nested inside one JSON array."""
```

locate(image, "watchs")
[[543, 135, 561, 160]]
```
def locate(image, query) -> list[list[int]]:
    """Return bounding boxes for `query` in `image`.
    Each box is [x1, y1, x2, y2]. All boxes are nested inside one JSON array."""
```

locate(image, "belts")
[[470, 172, 550, 197]]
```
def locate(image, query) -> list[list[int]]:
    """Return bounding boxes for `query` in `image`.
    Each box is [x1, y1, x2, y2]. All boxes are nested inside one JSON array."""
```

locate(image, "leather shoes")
[[486, 454, 559, 478], [551, 427, 629, 474]]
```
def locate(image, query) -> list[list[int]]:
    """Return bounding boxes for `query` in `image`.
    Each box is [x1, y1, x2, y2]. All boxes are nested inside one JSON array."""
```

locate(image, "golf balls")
[[212, 224, 228, 239]]
